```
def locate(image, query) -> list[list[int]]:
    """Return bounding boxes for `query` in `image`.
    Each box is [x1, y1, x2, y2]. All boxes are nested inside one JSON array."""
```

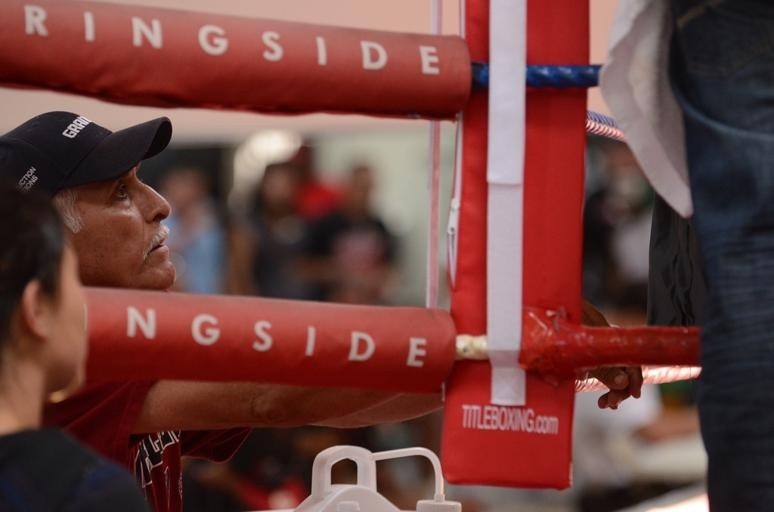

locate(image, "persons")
[[0, 182, 157, 511], [580, 122, 700, 442], [596, 0, 772, 512], [394, 360, 702, 511], [157, 149, 421, 512], [0, 108, 648, 511]]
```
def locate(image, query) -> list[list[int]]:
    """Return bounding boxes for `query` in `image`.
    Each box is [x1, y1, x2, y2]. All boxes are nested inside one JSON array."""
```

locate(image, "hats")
[[228, 127, 313, 211], [1, 111, 173, 203]]
